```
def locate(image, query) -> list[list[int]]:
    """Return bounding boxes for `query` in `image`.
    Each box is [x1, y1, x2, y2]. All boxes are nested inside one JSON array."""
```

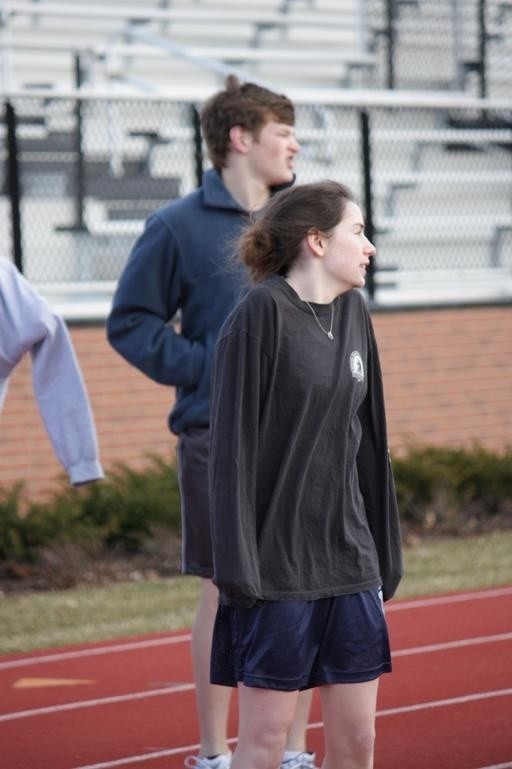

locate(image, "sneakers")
[[194, 749, 233, 769], [278, 751, 321, 769]]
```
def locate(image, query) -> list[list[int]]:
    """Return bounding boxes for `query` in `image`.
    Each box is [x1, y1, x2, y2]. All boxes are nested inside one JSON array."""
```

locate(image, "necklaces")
[[286, 278, 339, 345]]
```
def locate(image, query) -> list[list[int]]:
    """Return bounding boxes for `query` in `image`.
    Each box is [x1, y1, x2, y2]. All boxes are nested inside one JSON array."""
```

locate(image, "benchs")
[[1, 0, 511, 284]]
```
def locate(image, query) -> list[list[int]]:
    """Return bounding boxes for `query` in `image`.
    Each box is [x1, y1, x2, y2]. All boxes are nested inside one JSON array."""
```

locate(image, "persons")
[[0, 251, 106, 491], [204, 174, 409, 769], [101, 82, 318, 769]]
